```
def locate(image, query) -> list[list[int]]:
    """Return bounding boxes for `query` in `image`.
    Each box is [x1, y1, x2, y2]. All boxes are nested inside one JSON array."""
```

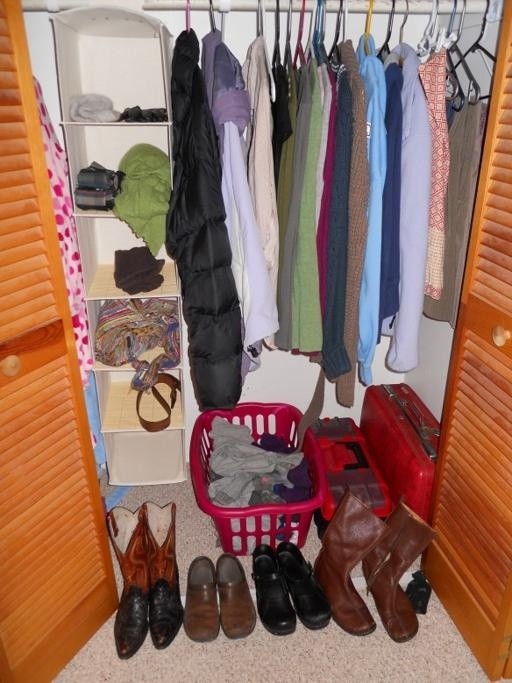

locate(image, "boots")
[[142, 501, 183, 650], [363, 500, 438, 642], [313, 491, 392, 635], [106, 506, 150, 658]]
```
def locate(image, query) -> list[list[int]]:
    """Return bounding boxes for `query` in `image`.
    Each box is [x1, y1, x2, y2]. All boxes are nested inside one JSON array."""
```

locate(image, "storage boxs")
[[309, 417, 392, 539]]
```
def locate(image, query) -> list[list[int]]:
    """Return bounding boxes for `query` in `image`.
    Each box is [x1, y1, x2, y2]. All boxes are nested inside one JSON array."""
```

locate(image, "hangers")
[[172, 0, 499, 105]]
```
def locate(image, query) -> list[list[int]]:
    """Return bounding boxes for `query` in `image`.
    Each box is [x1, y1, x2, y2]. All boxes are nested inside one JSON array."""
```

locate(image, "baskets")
[[190, 402, 328, 556]]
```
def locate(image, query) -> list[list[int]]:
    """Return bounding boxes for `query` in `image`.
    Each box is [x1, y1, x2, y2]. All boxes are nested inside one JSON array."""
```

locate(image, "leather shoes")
[[184, 557, 220, 642], [216, 554, 256, 639], [252, 544, 296, 635], [277, 541, 331, 630]]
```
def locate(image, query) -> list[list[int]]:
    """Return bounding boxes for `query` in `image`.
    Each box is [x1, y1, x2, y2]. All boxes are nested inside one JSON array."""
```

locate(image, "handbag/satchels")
[[93, 299, 180, 366]]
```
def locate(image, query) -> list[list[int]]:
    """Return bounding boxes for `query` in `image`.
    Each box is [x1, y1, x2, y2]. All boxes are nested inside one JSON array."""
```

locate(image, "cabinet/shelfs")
[[49, 8, 196, 488]]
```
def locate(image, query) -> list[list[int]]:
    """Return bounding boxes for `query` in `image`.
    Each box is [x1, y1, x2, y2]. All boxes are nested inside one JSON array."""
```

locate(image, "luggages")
[[360, 383, 441, 525]]
